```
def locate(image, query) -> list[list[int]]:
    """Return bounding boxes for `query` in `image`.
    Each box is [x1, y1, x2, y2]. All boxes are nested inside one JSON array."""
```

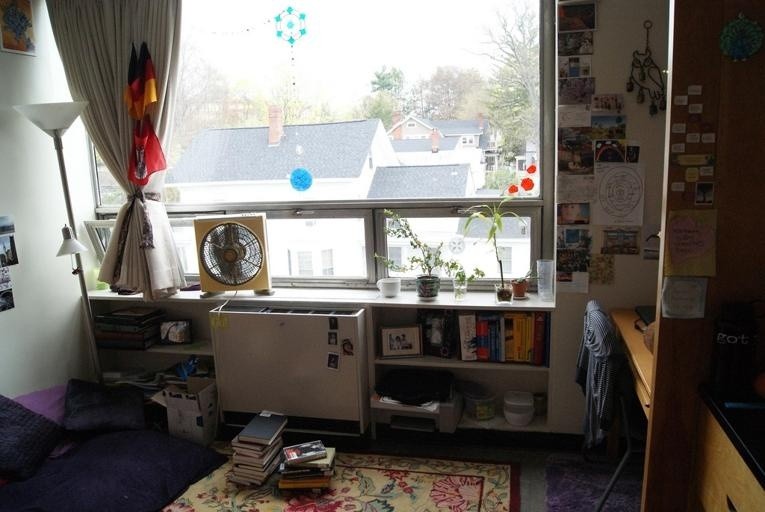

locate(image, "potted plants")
[[372, 208, 453, 301], [444, 261, 485, 299], [510, 277, 528, 297], [460, 195, 527, 301]]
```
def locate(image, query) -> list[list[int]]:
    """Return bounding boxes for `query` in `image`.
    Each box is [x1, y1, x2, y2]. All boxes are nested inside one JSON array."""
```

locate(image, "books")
[[226, 406, 337, 492], [457, 308, 551, 367], [93, 306, 170, 351], [369, 392, 441, 415]]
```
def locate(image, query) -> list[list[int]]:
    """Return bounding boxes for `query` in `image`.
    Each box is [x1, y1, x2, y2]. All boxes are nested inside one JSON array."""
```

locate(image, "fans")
[[193, 212, 275, 299]]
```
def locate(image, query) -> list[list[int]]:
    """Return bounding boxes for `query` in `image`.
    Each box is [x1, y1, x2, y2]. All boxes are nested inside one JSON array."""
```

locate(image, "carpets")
[[134, 438, 522, 512], [544, 456, 642, 512]]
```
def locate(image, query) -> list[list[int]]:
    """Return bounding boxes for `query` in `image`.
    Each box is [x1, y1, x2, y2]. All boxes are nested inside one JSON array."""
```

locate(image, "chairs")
[[584, 299, 645, 512]]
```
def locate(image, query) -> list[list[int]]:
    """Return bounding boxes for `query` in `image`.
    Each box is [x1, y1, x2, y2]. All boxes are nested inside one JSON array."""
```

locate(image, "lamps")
[[11, 100, 105, 389]]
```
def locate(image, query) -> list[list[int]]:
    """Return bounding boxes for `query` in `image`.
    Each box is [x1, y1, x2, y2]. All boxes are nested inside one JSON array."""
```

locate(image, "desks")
[[610, 307, 654, 465]]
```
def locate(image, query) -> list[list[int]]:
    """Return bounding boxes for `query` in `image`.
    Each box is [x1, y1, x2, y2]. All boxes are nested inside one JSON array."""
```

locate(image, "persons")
[[391, 336, 403, 350], [341, 338, 354, 355], [400, 333, 412, 348], [426, 317, 443, 345], [557, 204, 588, 225], [330, 317, 337, 328], [328, 355, 338, 368], [329, 332, 337, 344]]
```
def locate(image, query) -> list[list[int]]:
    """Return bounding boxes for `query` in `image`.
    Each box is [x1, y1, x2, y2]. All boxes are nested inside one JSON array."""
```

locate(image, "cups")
[[452, 278, 468, 301], [536, 259, 554, 302], [376, 278, 402, 297]]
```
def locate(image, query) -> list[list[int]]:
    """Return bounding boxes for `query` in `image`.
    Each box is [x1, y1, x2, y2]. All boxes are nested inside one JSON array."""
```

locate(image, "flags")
[[124, 41, 157, 120], [126, 116, 168, 186]]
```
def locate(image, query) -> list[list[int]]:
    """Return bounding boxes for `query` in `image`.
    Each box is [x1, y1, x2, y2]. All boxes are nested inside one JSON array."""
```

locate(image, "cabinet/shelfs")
[[82, 295, 552, 439], [688, 401, 764, 512]]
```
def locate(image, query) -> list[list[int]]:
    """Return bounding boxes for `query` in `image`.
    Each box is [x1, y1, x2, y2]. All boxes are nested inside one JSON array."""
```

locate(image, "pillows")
[[0, 394, 64, 483], [60, 377, 146, 432]]
